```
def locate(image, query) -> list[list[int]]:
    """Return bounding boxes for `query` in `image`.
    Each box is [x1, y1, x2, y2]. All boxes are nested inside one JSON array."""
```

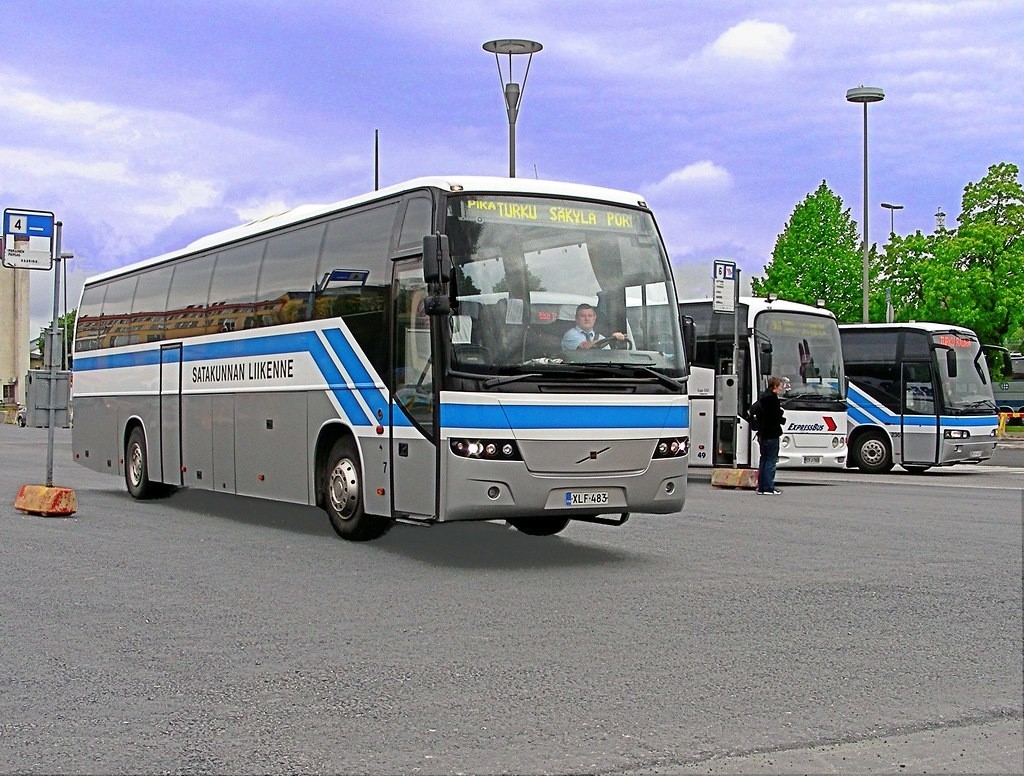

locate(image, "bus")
[[71, 176, 697, 542], [452, 293, 849, 470], [696, 318, 1024, 474]]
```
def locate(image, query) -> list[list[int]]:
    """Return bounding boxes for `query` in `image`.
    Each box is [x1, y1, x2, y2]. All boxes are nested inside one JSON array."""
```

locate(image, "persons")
[[561, 304, 627, 356], [941, 381, 959, 404], [756, 377, 786, 495]]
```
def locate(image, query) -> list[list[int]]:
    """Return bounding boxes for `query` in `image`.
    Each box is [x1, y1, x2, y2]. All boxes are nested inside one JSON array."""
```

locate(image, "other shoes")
[[757, 489, 782, 495]]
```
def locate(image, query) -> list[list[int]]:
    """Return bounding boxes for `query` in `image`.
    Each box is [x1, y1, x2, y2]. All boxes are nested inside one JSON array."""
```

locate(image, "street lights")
[[481, 39, 543, 177], [847, 82, 885, 324], [880, 204, 904, 244]]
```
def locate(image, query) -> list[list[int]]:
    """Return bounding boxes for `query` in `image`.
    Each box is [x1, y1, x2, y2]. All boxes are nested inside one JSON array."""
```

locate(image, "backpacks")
[[748, 393, 774, 430]]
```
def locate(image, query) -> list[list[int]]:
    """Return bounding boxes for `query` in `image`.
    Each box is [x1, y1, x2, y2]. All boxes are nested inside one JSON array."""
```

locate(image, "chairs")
[[458, 297, 534, 356]]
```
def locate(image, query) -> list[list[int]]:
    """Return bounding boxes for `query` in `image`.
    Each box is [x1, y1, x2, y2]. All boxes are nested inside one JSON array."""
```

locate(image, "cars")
[[17, 408, 27, 427]]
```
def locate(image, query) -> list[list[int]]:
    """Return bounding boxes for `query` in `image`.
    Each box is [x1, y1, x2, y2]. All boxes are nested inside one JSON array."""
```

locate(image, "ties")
[[581, 331, 593, 341]]
